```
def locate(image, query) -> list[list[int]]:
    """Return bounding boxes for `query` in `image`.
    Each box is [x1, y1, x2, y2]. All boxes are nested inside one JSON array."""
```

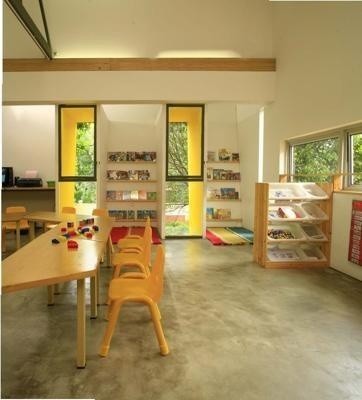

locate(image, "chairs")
[[1, 205, 108, 265], [99, 214, 171, 359]]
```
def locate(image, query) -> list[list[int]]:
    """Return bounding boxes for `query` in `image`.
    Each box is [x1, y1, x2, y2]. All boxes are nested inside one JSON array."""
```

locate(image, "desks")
[[2, 210, 114, 369]]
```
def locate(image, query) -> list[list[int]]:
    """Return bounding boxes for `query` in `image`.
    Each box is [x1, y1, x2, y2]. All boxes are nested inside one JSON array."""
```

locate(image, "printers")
[[15, 177, 43, 187]]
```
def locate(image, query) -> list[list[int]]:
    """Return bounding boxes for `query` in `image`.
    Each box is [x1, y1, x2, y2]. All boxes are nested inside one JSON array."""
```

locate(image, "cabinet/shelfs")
[[255, 183, 333, 269], [105, 160, 160, 227], [206, 161, 243, 222]]
